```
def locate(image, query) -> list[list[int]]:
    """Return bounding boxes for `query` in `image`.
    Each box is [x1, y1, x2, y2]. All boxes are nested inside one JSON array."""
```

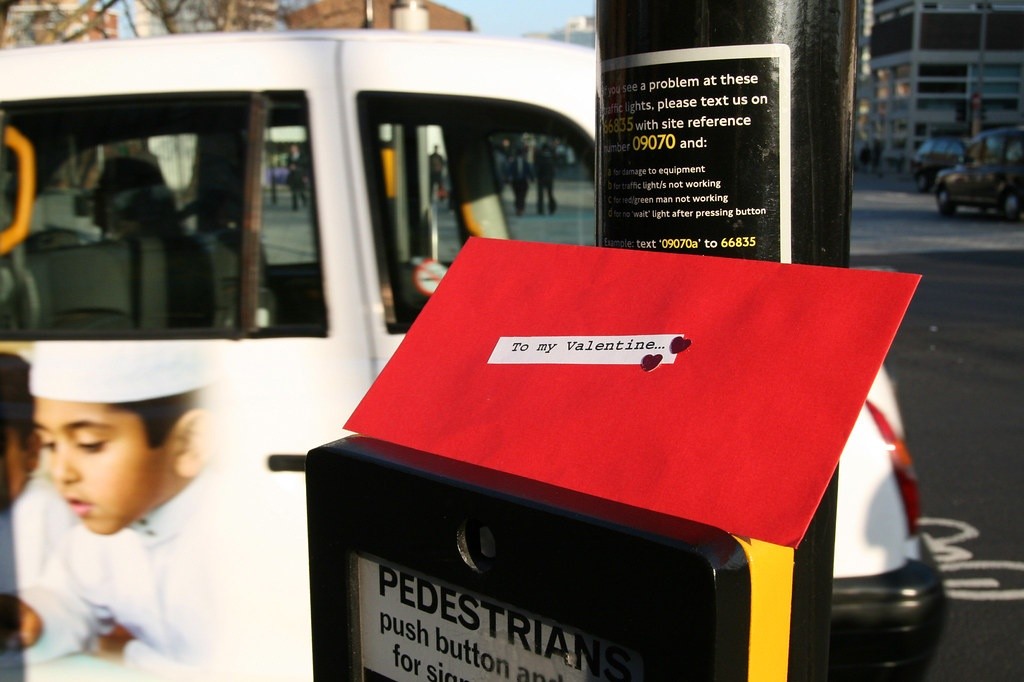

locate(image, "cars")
[[0, 29, 951, 682], [910, 138, 966, 193]]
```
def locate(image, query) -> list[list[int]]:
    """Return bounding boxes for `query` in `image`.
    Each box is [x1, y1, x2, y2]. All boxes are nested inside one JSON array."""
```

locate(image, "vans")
[[933, 122, 1024, 225]]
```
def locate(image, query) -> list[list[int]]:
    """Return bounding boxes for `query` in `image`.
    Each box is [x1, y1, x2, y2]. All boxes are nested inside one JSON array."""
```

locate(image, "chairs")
[[0, 220, 273, 333]]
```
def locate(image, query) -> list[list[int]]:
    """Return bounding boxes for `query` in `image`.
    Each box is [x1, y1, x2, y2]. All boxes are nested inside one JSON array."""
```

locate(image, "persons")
[[94, 156, 179, 242], [499, 135, 587, 215], [0, 340, 312, 667], [286, 144, 311, 210], [0, 351, 38, 512], [429, 145, 447, 203]]
